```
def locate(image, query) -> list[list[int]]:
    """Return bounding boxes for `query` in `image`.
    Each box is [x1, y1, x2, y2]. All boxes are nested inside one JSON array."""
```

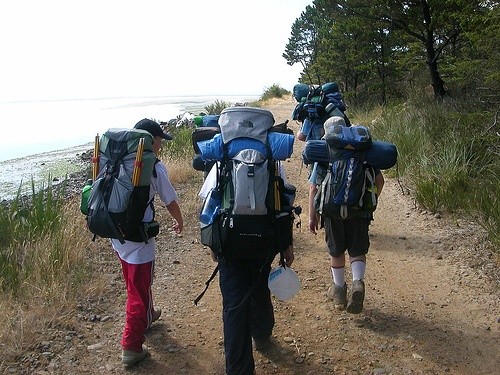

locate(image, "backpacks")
[[86, 129, 155, 244], [199, 107, 293, 252], [302, 84, 329, 122], [314, 125, 378, 220]]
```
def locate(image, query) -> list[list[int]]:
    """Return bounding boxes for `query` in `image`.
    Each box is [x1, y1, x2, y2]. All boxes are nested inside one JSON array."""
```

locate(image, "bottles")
[[200, 185, 223, 225], [364, 185, 378, 213], [268, 259, 301, 302], [325, 103, 345, 119], [285, 183, 296, 208], [81, 179, 93, 215]]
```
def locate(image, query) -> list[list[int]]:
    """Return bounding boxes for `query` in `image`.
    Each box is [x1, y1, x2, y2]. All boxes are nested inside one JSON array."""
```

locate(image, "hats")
[[134, 119, 173, 140], [324, 115, 346, 132]]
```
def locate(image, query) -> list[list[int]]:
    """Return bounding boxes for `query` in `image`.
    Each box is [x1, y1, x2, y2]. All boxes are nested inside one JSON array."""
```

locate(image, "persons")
[[198, 117, 384, 375], [107, 119, 183, 364]]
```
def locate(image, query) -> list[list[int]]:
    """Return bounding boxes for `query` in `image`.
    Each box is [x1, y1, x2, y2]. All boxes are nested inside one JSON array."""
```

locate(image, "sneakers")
[[121, 344, 149, 364], [327, 281, 347, 310], [145, 305, 161, 332], [346, 279, 365, 315]]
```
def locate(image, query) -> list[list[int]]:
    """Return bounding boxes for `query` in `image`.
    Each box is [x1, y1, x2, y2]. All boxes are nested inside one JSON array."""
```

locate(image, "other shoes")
[[255, 334, 270, 352]]
